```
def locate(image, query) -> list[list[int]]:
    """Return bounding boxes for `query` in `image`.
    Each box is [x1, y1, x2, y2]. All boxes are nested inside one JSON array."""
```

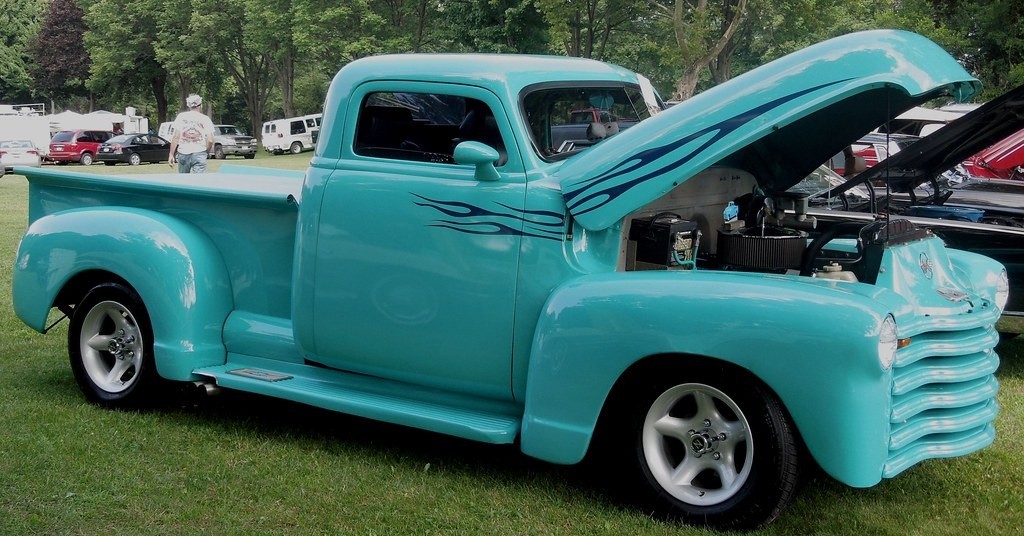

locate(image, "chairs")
[[356, 105, 415, 161], [454, 107, 505, 163]]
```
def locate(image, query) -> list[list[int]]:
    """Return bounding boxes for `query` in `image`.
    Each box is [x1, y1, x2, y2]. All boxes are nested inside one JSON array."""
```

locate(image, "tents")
[[45, 110, 149, 143]]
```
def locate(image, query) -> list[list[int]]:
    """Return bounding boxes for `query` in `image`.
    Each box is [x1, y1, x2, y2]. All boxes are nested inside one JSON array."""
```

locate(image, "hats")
[[186, 95, 202, 107]]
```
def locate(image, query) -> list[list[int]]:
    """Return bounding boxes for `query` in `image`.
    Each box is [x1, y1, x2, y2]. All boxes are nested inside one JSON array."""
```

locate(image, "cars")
[[550, 94, 1024, 332], [0, 103, 323, 176]]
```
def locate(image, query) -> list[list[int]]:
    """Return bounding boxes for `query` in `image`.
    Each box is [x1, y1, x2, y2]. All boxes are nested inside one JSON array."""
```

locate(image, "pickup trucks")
[[0, 28, 1010, 532]]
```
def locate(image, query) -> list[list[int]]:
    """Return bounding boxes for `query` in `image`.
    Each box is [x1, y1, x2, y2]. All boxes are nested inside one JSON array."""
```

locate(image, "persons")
[[168, 94, 214, 174], [116, 122, 122, 136]]
